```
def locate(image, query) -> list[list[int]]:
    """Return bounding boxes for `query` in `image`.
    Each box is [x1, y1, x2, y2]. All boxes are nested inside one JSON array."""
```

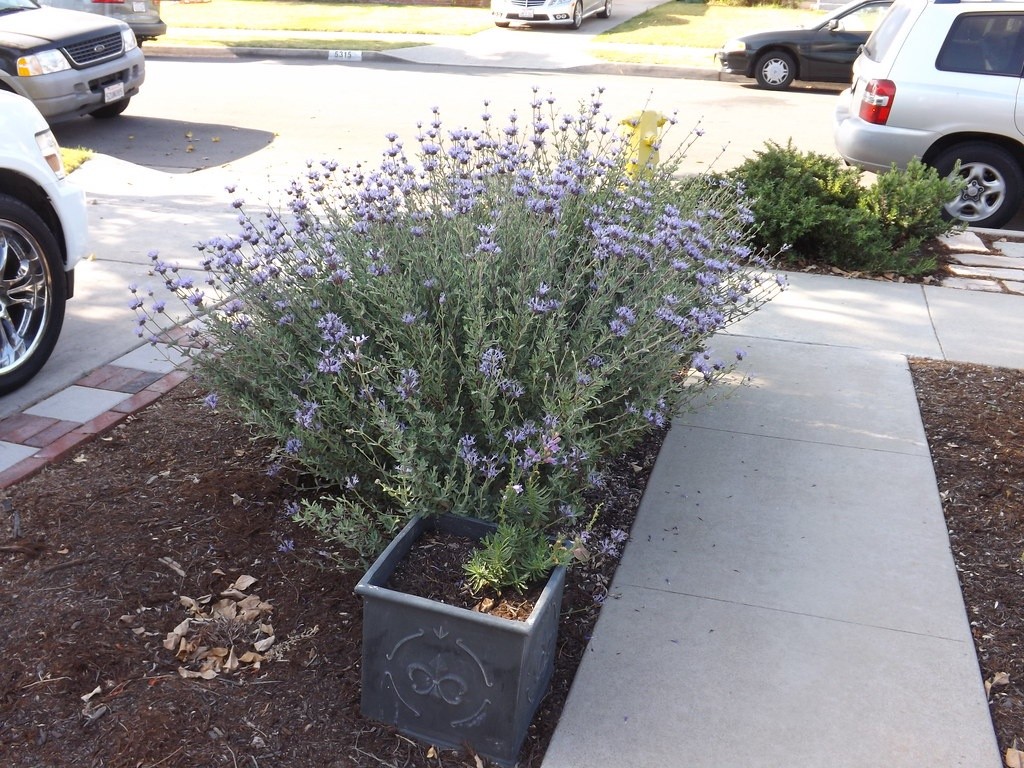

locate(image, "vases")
[[354, 506, 571, 767]]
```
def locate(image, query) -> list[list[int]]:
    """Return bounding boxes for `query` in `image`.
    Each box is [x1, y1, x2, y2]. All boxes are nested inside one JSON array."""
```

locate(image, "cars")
[[0, 91, 87, 395], [832, 1, 1024, 229], [491, 0, 613, 30], [36, 0, 167, 48], [718, 0, 897, 92], [1, 0, 145, 128]]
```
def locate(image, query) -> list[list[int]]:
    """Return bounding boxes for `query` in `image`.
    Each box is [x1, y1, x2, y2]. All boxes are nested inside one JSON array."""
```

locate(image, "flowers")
[[453, 429, 592, 598]]
